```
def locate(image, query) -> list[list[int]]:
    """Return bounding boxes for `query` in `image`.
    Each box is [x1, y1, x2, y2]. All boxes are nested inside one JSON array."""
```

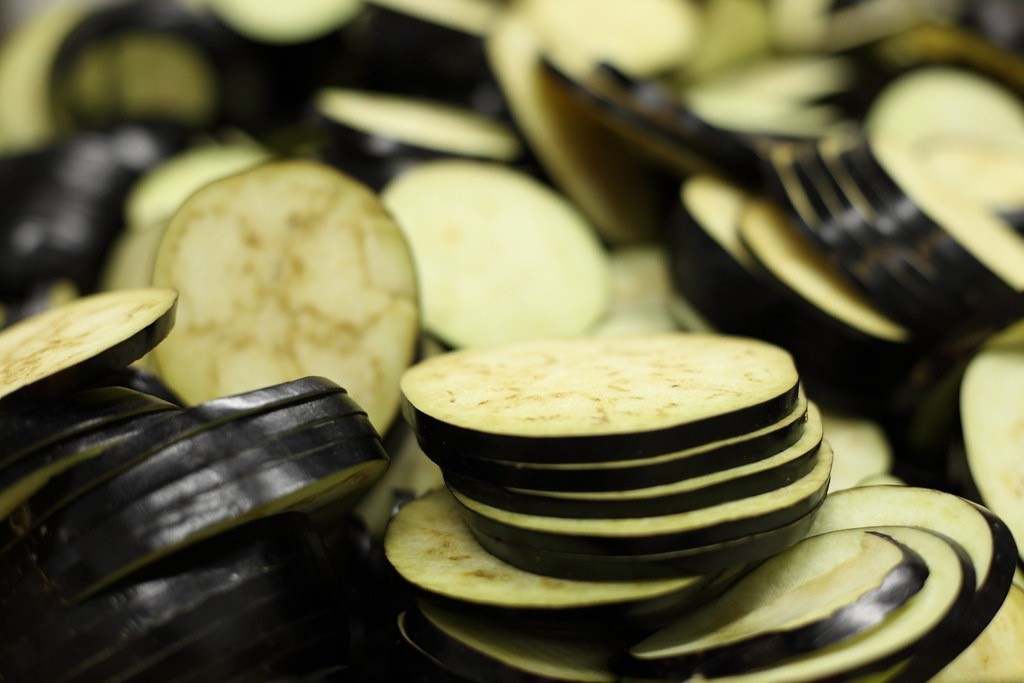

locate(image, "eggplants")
[[0, 0, 1024, 683]]
